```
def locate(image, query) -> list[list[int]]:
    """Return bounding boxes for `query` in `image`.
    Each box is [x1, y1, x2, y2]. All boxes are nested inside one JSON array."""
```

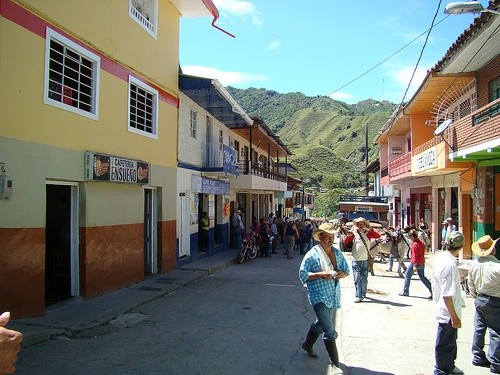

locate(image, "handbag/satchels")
[[368, 257, 373, 267]]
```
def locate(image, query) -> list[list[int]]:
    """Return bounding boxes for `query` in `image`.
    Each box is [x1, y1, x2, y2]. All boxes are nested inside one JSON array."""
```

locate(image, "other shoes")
[[448, 372, 459, 375], [355, 298, 362, 303], [452, 366, 463, 375], [429, 295, 432, 299], [399, 293, 409, 296]]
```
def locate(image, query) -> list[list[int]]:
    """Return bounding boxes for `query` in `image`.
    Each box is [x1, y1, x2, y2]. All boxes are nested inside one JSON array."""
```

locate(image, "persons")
[[441, 218, 459, 265], [232, 210, 242, 250], [335, 217, 430, 278], [398, 229, 432, 299], [252, 212, 318, 259], [468, 234, 500, 374], [0, 312, 23, 375], [200, 211, 210, 250], [299, 222, 350, 375], [351, 217, 371, 302], [431, 231, 464, 375]]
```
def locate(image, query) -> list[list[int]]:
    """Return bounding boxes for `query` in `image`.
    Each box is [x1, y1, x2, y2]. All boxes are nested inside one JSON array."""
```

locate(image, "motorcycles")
[[237, 226, 261, 264]]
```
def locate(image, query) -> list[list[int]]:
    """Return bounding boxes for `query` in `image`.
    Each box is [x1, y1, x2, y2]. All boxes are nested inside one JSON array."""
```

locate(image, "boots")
[[302, 328, 319, 357], [324, 339, 345, 375]]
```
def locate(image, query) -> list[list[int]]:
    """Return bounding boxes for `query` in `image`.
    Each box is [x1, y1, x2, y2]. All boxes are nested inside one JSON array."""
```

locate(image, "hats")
[[353, 217, 366, 229], [445, 231, 465, 247], [236, 210, 241, 214], [471, 235, 500, 257], [312, 223, 340, 241], [287, 217, 295, 221]]
